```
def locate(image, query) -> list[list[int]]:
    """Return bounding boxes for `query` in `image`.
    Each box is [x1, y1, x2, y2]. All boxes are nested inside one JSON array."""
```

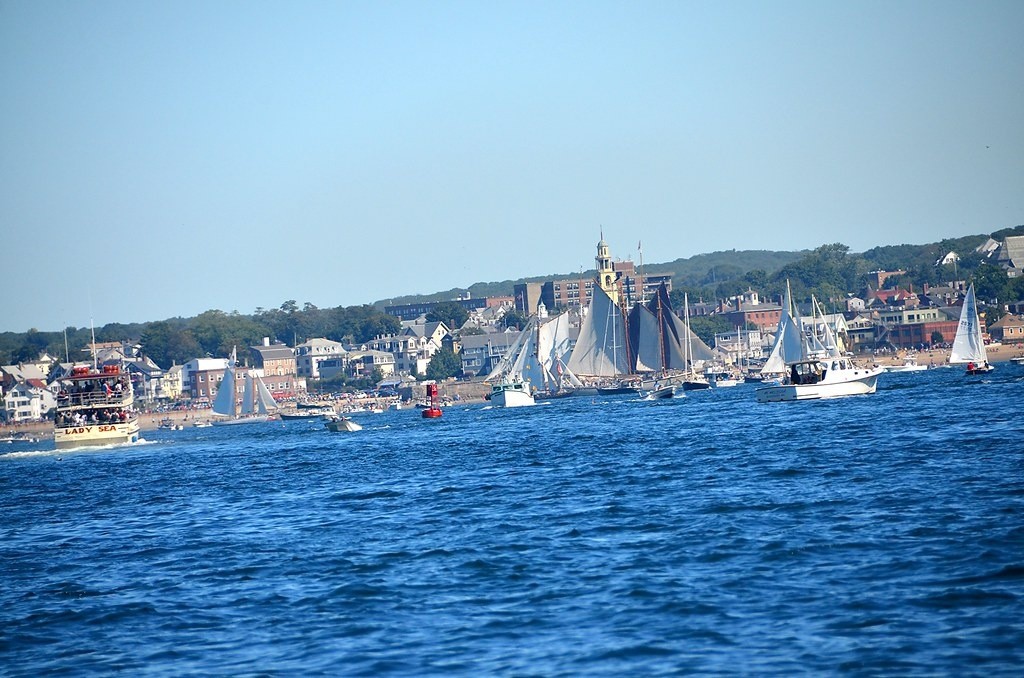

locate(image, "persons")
[[54, 363, 133, 428]]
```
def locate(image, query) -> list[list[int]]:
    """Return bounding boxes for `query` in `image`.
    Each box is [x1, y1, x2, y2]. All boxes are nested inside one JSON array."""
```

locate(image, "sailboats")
[[209, 345, 282, 425], [948, 282, 996, 376], [485, 269, 931, 404]]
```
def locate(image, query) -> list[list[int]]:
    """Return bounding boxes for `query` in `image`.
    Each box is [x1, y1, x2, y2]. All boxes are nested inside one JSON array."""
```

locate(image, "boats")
[[338, 401, 384, 416], [280, 401, 338, 421], [421, 385, 444, 418], [157, 417, 176, 432], [416, 402, 431, 409], [439, 399, 452, 408], [323, 417, 364, 433], [388, 400, 401, 412], [191, 421, 213, 429], [489, 375, 536, 408], [1010, 352, 1023, 367], [52, 317, 141, 449]]
